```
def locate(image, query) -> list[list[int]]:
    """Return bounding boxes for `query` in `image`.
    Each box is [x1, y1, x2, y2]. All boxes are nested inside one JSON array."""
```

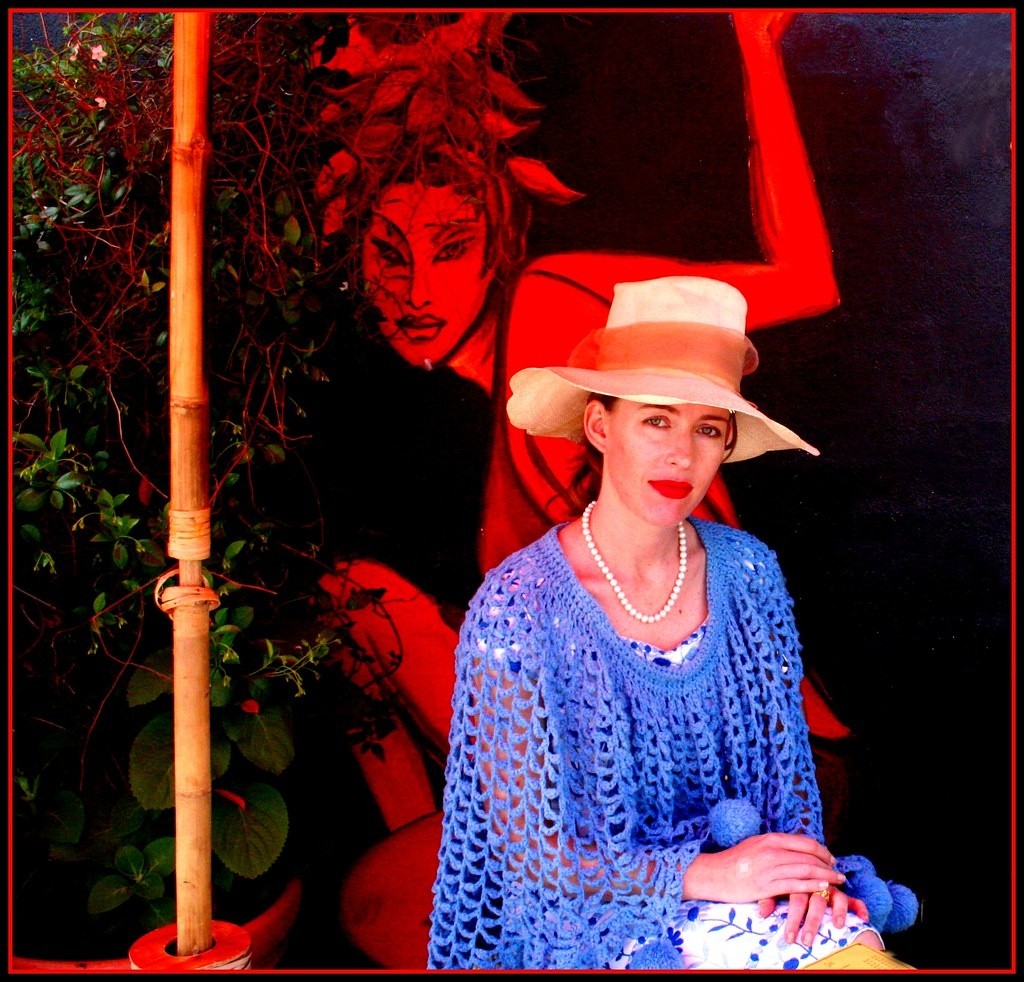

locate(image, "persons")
[[426, 274, 920, 970]]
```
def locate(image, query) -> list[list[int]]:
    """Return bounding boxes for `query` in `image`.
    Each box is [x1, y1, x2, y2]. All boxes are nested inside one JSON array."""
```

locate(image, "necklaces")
[[580, 502, 688, 623]]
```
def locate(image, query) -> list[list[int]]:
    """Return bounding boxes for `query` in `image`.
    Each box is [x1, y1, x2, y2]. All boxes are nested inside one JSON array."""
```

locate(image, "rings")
[[812, 889, 830, 901]]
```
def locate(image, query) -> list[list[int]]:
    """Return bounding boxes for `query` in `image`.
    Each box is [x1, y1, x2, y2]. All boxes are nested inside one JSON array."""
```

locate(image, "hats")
[[505, 276, 821, 464]]
